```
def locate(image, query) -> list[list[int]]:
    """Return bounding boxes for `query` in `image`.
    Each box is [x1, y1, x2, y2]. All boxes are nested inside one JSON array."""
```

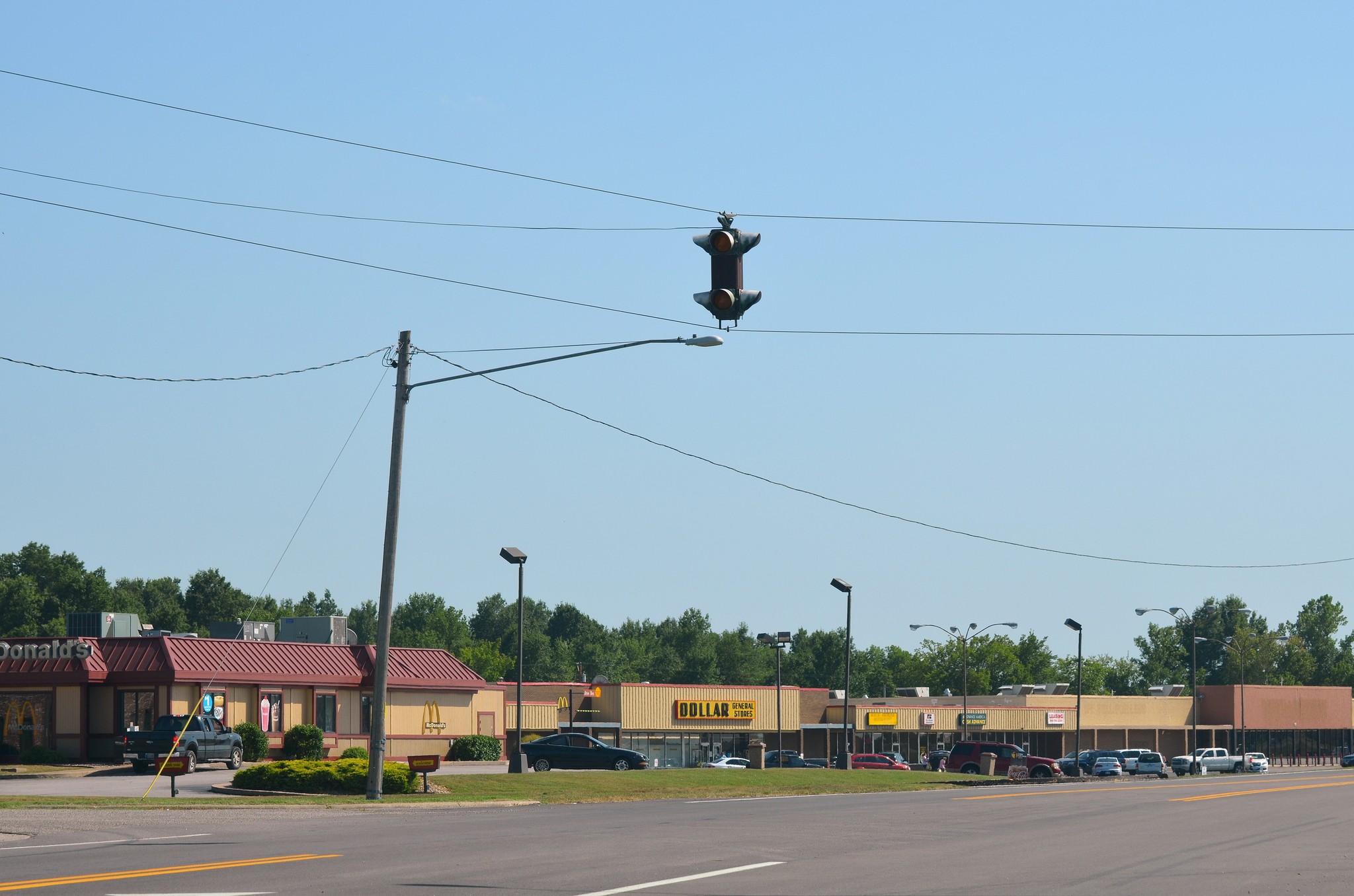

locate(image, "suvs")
[[926, 750, 951, 771], [944, 740, 1061, 779], [1055, 748, 1127, 770], [1115, 747, 1170, 775], [850, 751, 912, 771]]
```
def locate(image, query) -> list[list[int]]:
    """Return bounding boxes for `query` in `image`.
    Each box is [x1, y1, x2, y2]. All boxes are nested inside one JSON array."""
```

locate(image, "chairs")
[[172, 721, 182, 729]]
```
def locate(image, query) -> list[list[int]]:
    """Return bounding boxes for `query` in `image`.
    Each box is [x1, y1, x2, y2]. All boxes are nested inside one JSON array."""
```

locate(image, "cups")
[[261, 704, 270, 732], [272, 712, 280, 732]]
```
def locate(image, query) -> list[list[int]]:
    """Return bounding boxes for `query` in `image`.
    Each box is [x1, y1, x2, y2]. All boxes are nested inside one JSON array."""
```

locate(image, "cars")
[[1341, 753, 1354, 767], [519, 732, 649, 773], [1245, 752, 1269, 773], [702, 757, 750, 769], [1090, 757, 1123, 777]]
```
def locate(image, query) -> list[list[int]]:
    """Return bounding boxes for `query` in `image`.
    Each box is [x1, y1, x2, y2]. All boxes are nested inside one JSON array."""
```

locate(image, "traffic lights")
[[694, 226, 766, 325]]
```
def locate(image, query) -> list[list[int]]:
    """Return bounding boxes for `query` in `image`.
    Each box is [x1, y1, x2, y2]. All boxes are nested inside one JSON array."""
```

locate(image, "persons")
[[719, 752, 726, 758], [922, 753, 928, 769], [929, 751, 933, 759], [939, 757, 947, 771]]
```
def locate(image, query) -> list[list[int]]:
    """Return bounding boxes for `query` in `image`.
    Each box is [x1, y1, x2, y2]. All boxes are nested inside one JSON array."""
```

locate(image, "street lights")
[[756, 631, 791, 768], [1064, 618, 1084, 778], [1136, 604, 1289, 773], [366, 328, 723, 800], [831, 577, 853, 770], [909, 620, 1018, 738], [499, 547, 529, 772]]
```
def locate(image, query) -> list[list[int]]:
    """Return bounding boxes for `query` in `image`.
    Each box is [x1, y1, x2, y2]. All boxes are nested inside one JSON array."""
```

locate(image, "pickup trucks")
[[123, 715, 245, 774], [764, 750, 830, 768], [1171, 748, 1253, 777]]
